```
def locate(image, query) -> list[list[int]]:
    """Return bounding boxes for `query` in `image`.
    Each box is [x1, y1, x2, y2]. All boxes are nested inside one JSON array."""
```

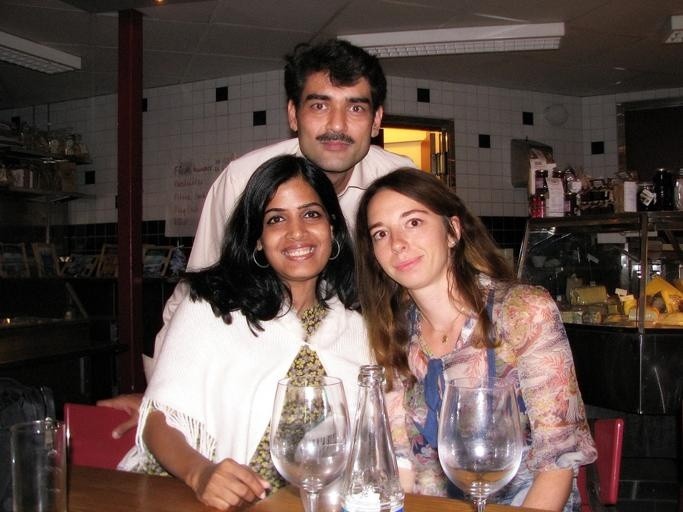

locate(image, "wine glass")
[[269, 375, 352, 511], [437, 376, 522, 511]]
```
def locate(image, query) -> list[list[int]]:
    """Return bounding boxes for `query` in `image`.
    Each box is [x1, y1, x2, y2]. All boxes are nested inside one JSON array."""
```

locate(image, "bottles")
[[338, 365, 406, 511], [654, 168, 674, 211]]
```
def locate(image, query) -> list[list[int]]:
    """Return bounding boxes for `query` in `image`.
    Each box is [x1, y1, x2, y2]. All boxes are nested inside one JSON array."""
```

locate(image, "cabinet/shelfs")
[[0, 135, 97, 244], [515, 212, 683, 460]]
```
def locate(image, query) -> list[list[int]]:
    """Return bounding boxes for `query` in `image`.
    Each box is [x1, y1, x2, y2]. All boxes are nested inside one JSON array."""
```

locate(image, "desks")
[[55, 463, 555, 511]]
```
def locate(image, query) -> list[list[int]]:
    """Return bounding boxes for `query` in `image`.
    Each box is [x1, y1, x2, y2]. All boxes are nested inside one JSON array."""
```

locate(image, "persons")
[[96, 39, 416, 440], [116, 154, 367, 512], [356, 168, 597, 512]]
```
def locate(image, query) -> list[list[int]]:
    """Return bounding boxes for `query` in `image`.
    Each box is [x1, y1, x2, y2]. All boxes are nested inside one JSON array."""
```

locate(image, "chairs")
[[55, 403, 138, 471], [524, 417, 623, 512]]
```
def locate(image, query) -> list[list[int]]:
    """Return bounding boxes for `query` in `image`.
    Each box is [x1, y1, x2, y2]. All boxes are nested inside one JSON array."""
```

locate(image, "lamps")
[[0, 31, 82, 75], [661, 15, 683, 44], [337, 21, 566, 60]]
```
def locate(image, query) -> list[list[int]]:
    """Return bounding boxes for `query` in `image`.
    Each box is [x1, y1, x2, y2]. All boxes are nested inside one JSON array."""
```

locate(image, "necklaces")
[[421, 309, 465, 344]]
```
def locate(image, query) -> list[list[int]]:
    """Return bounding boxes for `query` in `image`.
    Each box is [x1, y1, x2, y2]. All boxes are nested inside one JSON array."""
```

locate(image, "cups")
[[10, 419, 68, 512]]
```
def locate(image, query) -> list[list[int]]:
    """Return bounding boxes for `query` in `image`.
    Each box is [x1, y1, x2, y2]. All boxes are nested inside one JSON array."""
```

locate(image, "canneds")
[[535, 169, 548, 194], [530, 195, 544, 218], [554, 168, 565, 186], [638, 183, 654, 211], [565, 192, 577, 215]]
